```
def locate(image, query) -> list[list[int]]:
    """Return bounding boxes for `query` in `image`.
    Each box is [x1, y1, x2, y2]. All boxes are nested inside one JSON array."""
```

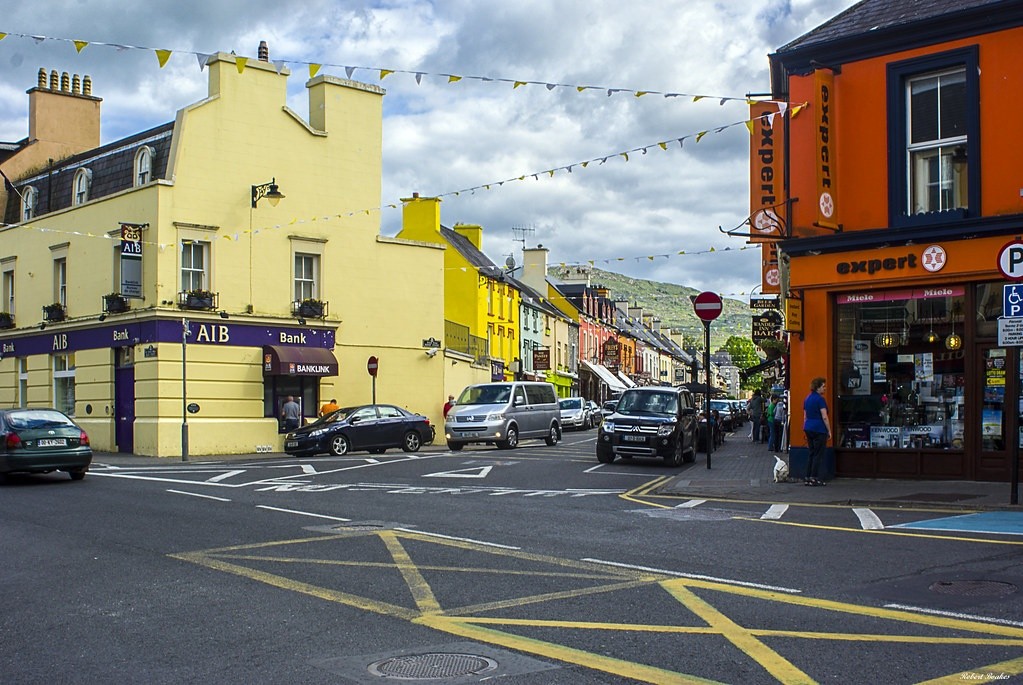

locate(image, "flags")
[[814, 65, 839, 232], [749, 99, 787, 245]]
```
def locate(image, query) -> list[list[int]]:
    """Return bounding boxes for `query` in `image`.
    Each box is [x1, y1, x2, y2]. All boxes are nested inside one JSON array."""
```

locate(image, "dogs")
[[772, 454, 790, 484]]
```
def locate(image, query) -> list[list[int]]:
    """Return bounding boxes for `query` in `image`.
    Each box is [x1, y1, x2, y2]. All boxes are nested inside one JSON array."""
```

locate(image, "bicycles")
[[423, 424, 435, 446]]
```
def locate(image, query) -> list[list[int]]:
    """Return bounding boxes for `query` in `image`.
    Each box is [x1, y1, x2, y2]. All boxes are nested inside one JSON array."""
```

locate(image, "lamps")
[[945, 297, 961, 349], [875, 300, 900, 348], [298, 315, 306, 326], [40, 322, 50, 330], [922, 297, 941, 343], [220, 310, 229, 318], [99, 313, 110, 321], [951, 145, 968, 172], [250, 176, 286, 208], [900, 300, 910, 346]]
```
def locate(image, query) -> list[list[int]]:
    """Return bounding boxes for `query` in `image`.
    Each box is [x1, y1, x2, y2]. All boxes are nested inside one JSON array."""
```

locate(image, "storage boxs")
[[844, 374, 965, 451]]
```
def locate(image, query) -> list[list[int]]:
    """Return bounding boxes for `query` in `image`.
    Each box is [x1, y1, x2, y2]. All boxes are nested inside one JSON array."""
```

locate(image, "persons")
[[748, 386, 769, 444], [281, 396, 300, 434], [443, 395, 455, 419], [698, 409, 724, 450], [803, 377, 833, 486], [766, 393, 785, 452], [318, 399, 339, 418]]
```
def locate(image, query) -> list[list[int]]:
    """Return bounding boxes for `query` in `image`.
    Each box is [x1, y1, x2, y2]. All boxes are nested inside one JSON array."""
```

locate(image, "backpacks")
[[760, 411, 770, 425]]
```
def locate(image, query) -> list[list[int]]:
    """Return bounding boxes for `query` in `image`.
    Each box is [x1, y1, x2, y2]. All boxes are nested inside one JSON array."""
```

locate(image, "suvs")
[[594, 386, 701, 469]]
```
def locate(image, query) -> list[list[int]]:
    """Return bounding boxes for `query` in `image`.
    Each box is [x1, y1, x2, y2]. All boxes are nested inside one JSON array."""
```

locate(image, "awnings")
[[581, 359, 638, 393], [262, 344, 338, 376], [738, 359, 776, 382]]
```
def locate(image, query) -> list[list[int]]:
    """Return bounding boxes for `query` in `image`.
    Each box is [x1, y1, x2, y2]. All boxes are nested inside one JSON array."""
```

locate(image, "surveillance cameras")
[[426, 347, 438, 357]]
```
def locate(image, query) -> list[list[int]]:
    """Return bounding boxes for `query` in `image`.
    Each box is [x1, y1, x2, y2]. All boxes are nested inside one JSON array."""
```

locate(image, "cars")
[[282, 403, 434, 456], [0, 406, 93, 481], [696, 397, 752, 453], [558, 396, 626, 432]]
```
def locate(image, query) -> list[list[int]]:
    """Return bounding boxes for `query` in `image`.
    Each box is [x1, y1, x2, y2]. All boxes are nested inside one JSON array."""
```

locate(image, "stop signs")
[[367, 355, 378, 375], [693, 291, 723, 321]]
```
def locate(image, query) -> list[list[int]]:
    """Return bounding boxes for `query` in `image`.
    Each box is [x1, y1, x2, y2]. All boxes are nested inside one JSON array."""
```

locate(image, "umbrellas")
[[675, 381, 728, 407]]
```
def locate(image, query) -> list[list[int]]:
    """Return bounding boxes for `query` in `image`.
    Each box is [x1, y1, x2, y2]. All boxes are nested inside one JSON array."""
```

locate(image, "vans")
[[444, 381, 563, 451]]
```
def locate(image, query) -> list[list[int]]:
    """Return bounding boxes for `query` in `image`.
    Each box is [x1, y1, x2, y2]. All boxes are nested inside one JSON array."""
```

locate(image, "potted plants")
[[43, 302, 65, 320], [186, 288, 213, 308], [0, 312, 12, 328], [757, 339, 786, 358], [103, 292, 126, 311], [300, 298, 323, 316]]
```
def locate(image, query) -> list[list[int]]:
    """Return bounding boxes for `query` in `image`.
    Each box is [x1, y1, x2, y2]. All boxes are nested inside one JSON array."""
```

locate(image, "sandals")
[[805, 477, 827, 486]]
